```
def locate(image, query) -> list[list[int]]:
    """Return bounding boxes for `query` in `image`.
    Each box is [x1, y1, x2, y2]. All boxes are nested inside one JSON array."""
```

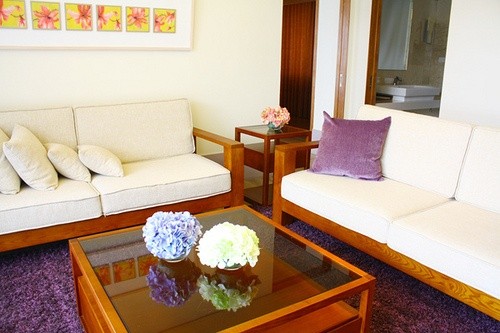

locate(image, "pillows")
[[305, 110, 392, 182], [76, 144, 124, 178], [0, 128, 21, 195], [2, 124, 59, 192], [44, 143, 92, 184]]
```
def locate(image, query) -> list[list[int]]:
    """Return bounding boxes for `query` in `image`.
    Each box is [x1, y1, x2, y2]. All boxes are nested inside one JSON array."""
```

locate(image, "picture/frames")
[[31, 1, 62, 30], [64, 3, 93, 30], [152, 8, 177, 33], [96, 5, 122, 32], [0, 0, 27, 28], [125, 7, 151, 33]]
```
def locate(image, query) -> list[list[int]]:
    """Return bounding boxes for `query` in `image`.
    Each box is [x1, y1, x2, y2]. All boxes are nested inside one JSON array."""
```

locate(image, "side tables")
[[235, 124, 313, 209]]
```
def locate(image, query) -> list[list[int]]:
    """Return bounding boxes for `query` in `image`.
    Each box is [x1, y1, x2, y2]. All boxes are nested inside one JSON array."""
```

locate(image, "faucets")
[[392, 76, 403, 86]]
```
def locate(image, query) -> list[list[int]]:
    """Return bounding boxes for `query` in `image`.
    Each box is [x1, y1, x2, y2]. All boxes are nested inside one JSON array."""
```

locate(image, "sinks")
[[376, 84, 440, 102]]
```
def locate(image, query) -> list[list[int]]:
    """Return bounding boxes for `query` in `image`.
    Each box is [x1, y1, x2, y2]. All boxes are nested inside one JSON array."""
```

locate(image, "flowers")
[[195, 221, 260, 269], [143, 211, 203, 261], [195, 268, 262, 313], [143, 257, 204, 308], [260, 105, 291, 135]]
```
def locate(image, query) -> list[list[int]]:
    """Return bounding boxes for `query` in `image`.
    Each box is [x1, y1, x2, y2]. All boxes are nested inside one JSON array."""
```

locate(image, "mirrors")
[[364, 0, 452, 118]]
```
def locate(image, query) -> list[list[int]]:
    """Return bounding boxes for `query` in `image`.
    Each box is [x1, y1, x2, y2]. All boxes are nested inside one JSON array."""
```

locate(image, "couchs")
[[271, 103, 500, 322], [0, 98, 245, 253]]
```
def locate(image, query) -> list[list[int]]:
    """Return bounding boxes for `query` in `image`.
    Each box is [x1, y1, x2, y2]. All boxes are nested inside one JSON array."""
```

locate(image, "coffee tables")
[[68, 204, 377, 333]]
